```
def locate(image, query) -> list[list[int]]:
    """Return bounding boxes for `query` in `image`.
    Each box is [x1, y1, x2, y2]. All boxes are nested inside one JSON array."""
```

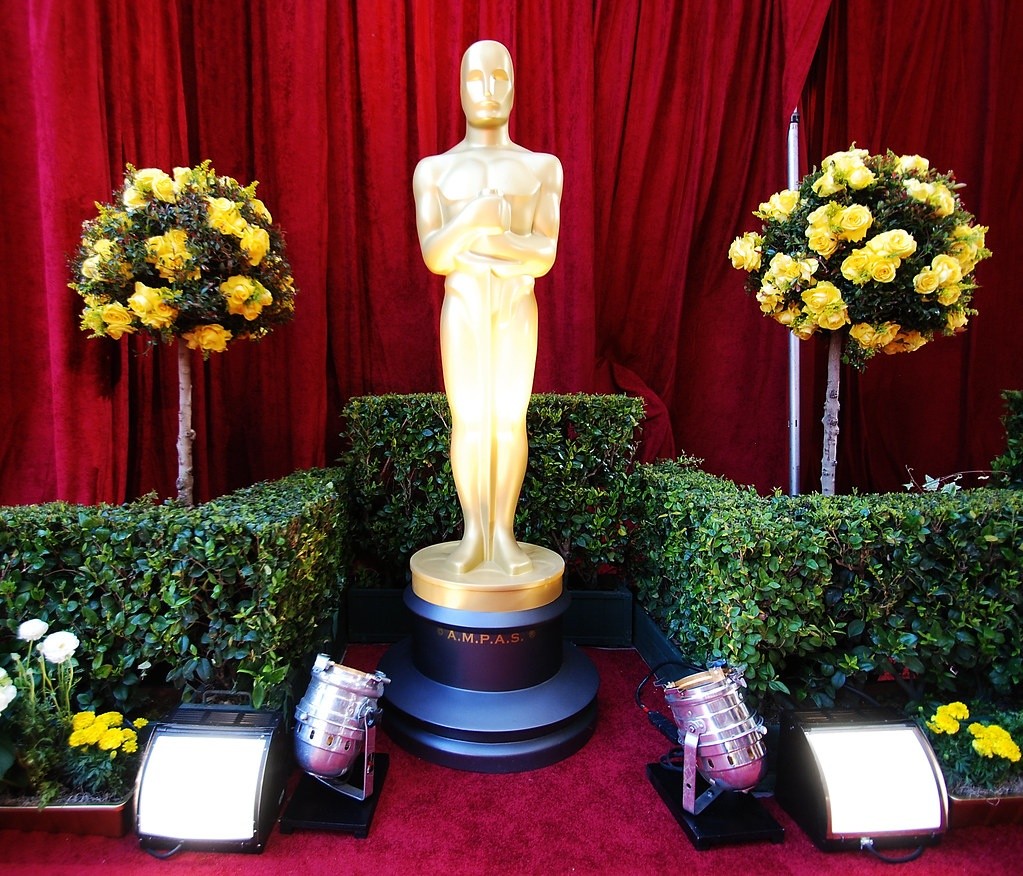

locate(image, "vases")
[[946, 780, 1023, 830], [0, 759, 135, 838]]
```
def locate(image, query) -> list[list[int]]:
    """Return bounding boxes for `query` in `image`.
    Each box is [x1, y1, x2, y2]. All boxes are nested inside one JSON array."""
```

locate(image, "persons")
[[413, 40, 564, 578]]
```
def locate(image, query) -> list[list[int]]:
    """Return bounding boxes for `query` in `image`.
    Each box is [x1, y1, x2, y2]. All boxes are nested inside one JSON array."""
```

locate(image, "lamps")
[[291, 653, 392, 801], [133, 688, 286, 854], [660, 665, 767, 815], [776, 706, 952, 844]]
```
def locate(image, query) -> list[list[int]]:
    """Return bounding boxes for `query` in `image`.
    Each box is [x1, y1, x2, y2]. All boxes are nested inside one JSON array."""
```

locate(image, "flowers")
[[727, 143, 992, 374], [0, 619, 148, 812], [904, 699, 1023, 792], [67, 159, 299, 361]]
